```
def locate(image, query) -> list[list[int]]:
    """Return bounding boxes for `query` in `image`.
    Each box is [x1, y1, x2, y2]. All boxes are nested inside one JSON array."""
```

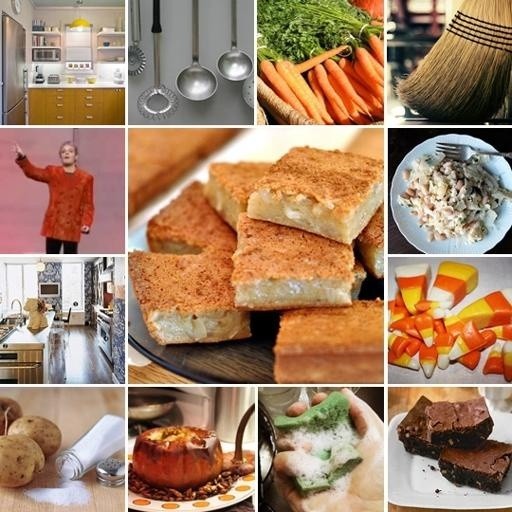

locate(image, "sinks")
[[1, 317, 27, 328]]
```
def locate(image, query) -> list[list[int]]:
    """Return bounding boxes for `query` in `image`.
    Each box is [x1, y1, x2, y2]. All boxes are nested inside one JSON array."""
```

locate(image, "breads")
[[128, 146, 384, 384]]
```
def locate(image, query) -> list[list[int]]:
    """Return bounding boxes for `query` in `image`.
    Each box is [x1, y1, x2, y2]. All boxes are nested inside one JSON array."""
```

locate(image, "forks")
[[434, 142, 512, 162]]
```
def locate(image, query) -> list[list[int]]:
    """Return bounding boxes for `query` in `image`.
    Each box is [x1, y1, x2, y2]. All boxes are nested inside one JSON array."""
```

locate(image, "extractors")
[[99, 258, 113, 282]]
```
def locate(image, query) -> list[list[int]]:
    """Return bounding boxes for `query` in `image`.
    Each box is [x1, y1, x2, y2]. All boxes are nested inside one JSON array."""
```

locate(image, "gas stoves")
[[99, 309, 114, 324]]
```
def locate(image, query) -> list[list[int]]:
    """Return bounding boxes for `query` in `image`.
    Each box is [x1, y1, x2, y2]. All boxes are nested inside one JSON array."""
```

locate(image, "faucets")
[[12, 299, 23, 316]]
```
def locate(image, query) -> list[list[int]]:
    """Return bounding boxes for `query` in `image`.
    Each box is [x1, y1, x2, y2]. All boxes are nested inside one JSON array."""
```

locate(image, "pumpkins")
[[132, 426, 224, 491]]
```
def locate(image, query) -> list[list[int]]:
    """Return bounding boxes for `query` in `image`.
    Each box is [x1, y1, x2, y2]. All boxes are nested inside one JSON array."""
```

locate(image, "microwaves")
[[33, 49, 60, 61]]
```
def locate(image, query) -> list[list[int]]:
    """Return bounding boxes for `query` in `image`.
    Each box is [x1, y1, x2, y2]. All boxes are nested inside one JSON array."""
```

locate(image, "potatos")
[[0, 397, 62, 488]]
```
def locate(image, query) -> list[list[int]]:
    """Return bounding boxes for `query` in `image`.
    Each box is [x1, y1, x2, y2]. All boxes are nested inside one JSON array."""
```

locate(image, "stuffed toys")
[[23, 298, 49, 330]]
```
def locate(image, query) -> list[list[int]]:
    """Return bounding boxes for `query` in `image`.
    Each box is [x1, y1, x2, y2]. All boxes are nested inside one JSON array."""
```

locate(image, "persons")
[[272, 387, 384, 512], [14, 142, 94, 254]]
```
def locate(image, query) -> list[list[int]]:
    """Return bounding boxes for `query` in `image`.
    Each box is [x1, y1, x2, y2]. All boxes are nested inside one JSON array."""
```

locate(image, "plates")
[[128, 128, 383, 384], [389, 133, 512, 254], [128, 433, 255, 512], [389, 409, 512, 511]]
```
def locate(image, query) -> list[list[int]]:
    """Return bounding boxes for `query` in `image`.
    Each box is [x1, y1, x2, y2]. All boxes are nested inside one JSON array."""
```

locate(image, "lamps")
[[35, 258, 45, 271], [69, 0, 91, 31]]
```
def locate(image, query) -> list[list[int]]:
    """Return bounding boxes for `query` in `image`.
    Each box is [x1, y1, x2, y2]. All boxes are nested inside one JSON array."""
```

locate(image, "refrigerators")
[[0, 9, 27, 125]]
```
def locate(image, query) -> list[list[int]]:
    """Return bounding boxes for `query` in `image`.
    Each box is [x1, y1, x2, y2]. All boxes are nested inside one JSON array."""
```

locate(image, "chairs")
[[62, 307, 71, 332]]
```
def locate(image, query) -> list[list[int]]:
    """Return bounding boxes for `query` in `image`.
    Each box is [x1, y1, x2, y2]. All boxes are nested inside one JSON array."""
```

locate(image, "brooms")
[[393, 0, 512, 125]]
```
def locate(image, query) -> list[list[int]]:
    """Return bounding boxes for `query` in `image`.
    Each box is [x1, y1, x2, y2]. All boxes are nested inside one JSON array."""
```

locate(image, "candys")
[[388, 261, 512, 385]]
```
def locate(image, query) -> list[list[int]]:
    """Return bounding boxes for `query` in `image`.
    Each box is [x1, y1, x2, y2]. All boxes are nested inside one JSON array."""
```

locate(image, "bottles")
[[54, 413, 126, 483]]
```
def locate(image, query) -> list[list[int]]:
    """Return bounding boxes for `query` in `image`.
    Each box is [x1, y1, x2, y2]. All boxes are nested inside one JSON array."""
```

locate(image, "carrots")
[[259, 35, 384, 126]]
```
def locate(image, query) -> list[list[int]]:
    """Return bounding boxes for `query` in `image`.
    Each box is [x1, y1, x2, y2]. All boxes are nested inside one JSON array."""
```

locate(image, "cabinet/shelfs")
[[96, 31, 125, 64], [28, 88, 125, 125], [31, 31, 62, 64]]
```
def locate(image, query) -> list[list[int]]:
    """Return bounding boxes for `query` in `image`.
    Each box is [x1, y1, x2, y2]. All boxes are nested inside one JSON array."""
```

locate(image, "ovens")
[[1, 345, 44, 383], [93, 314, 112, 364]]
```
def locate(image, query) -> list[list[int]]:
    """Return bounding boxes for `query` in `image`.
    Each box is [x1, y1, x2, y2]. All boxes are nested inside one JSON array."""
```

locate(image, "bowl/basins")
[[64, 75, 96, 84], [97, 56, 124, 61], [32, 25, 60, 31], [103, 41, 122, 46]]
